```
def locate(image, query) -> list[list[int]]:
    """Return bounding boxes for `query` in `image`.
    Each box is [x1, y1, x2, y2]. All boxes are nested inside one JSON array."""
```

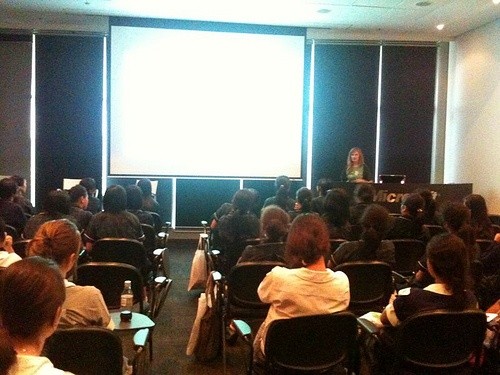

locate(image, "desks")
[[320, 179, 473, 215]]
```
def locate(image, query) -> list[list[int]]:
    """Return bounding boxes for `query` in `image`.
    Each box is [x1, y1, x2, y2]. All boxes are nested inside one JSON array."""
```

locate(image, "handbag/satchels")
[[184, 271, 216, 359], [194, 281, 221, 363], [187, 236, 211, 292]]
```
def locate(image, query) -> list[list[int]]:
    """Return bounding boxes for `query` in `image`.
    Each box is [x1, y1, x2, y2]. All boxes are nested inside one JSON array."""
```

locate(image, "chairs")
[[200, 211, 500, 375], [377, 174, 407, 184], [1, 212, 174, 375]]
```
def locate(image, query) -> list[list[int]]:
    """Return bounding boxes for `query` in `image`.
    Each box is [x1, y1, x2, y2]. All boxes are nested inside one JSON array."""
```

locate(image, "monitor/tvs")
[[379, 175, 405, 184]]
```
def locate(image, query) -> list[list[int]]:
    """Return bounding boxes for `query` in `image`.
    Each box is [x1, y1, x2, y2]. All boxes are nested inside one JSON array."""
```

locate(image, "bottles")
[[119, 280, 134, 322]]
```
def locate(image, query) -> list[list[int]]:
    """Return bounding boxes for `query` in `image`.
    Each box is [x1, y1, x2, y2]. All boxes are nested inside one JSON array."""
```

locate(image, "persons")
[[209, 175, 500, 375], [0, 176, 160, 375], [341, 147, 373, 183]]
